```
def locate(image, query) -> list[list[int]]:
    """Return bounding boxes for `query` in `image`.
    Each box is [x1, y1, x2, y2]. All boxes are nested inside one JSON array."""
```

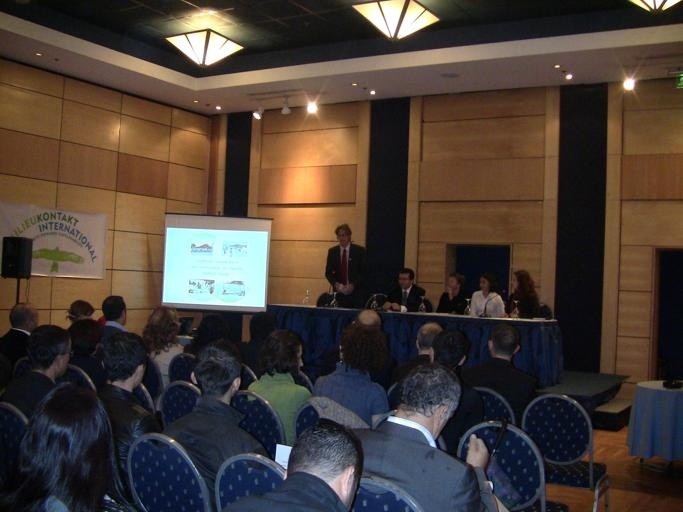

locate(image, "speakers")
[[1, 236, 32, 279]]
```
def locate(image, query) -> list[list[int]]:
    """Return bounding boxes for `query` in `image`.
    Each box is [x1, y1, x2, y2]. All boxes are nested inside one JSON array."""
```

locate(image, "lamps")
[[164, 28, 249, 73], [351, 1, 441, 43], [629, 1, 683, 19], [244, 93, 294, 121]]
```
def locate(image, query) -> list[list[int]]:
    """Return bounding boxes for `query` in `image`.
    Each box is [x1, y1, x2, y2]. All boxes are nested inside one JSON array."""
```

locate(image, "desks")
[[250, 301, 563, 385], [623, 379, 683, 471]]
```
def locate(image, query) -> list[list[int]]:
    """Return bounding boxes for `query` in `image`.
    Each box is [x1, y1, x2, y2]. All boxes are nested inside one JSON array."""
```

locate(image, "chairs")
[[239, 360, 257, 389], [293, 368, 315, 399], [130, 378, 155, 421], [213, 453, 291, 510], [57, 362, 97, 396], [387, 381, 411, 412], [370, 411, 448, 455], [126, 433, 210, 510], [0, 401, 29, 455], [350, 476, 426, 512], [453, 387, 516, 425], [159, 381, 202, 431], [454, 419, 571, 511], [522, 394, 611, 511], [229, 389, 287, 460]]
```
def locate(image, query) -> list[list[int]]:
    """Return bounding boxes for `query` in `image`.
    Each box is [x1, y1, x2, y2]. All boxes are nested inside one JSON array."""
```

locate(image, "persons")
[[326, 223, 369, 309], [382, 268, 541, 318], [1, 296, 538, 512]]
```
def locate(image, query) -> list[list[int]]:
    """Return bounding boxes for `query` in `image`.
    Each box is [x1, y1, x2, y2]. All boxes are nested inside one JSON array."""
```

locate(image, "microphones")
[[320, 271, 505, 317]]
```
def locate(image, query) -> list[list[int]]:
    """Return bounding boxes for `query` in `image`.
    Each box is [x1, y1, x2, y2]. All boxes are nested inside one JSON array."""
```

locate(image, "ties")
[[402, 291, 407, 303], [340, 250, 347, 284]]
